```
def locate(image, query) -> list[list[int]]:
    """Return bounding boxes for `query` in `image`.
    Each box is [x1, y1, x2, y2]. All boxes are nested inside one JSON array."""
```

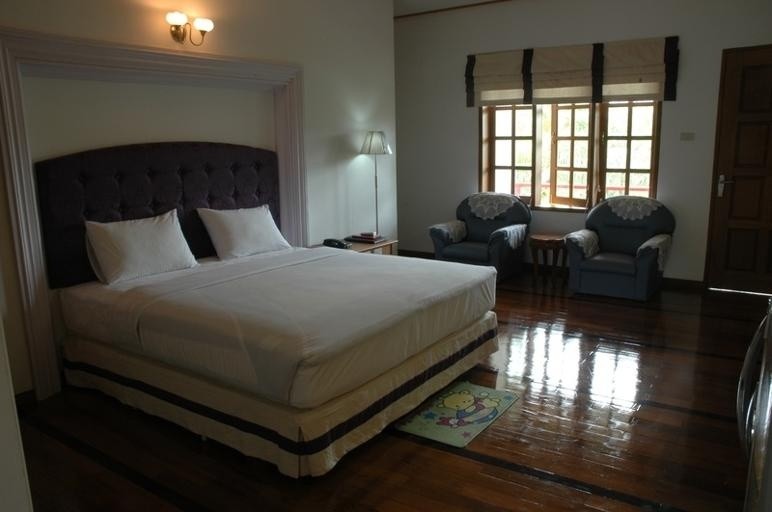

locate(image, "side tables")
[[528, 232, 567, 290]]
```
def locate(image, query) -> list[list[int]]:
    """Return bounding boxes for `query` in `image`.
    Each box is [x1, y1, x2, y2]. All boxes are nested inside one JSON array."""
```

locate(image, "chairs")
[[565, 196, 676, 303], [429, 192, 531, 285]]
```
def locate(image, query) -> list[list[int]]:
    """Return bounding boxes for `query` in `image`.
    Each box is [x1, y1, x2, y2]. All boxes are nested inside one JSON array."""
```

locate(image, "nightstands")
[[314, 239, 399, 253]]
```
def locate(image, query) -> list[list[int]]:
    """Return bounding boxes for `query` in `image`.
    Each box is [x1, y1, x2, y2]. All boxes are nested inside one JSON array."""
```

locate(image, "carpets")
[[395, 382, 520, 449]]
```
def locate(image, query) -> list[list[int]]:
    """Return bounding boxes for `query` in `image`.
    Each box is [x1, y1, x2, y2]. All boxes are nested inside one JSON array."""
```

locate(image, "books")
[[344, 235, 384, 243], [352, 234, 382, 239], [360, 232, 376, 236]]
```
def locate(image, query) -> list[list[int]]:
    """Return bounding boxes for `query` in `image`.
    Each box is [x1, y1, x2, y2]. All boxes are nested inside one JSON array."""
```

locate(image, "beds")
[[33, 141, 499, 481]]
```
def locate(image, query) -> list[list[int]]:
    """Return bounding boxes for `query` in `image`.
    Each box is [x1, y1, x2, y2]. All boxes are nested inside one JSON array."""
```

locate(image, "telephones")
[[323, 239, 352, 249]]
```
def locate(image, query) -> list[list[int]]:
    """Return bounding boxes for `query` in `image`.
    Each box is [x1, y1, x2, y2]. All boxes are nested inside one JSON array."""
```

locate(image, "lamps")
[[166, 11, 214, 46], [359, 131, 393, 236]]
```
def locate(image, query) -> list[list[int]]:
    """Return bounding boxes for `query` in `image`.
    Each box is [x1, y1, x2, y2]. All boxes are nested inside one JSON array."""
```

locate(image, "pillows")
[[197, 204, 292, 262], [84, 208, 199, 285]]
